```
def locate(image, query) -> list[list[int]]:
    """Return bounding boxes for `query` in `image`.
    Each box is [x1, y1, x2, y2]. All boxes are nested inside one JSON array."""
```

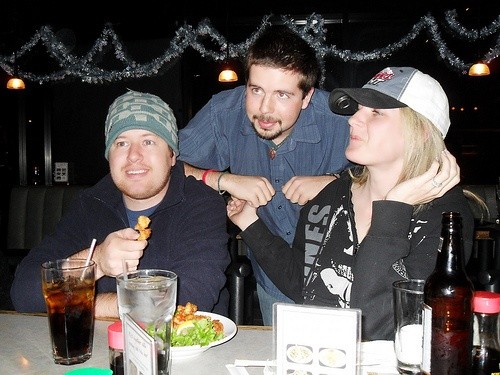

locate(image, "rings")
[[428, 179, 442, 187]]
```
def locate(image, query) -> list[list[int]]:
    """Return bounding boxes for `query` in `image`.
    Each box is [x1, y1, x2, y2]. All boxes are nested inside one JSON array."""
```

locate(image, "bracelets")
[[218, 171, 229, 195], [202, 169, 216, 183]]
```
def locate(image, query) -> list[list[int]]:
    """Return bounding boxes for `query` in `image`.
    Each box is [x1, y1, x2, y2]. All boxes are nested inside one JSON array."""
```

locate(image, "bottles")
[[422, 210, 476, 375]]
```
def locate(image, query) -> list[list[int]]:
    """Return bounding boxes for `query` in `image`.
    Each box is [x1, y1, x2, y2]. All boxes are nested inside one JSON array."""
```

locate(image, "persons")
[[10, 91, 230, 317], [178, 26, 358, 327], [225, 66, 473, 341]]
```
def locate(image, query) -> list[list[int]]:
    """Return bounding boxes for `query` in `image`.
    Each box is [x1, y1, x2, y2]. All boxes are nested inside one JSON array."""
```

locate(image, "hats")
[[105, 91, 180, 161], [328, 66, 451, 140]]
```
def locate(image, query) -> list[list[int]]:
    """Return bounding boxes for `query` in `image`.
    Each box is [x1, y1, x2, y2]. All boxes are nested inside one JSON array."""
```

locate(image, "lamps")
[[468, 0, 491, 77], [6, 0, 25, 90], [217, 0, 238, 82]]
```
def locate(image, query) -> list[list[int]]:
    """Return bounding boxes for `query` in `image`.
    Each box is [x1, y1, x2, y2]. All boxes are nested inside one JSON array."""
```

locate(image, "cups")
[[108, 321, 145, 375], [116, 269, 178, 375], [40, 258, 98, 366], [466, 290, 499, 374], [392, 279, 435, 374]]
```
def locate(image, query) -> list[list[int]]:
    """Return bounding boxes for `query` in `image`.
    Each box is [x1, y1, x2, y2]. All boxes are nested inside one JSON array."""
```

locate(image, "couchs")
[[0, 179, 500, 325]]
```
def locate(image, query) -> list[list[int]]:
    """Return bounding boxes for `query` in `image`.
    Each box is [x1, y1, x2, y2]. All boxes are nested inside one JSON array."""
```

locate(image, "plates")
[[163, 310, 237, 358]]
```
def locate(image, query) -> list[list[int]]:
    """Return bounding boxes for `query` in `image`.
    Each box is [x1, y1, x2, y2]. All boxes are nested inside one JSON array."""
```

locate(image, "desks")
[[0, 310, 399, 375]]
